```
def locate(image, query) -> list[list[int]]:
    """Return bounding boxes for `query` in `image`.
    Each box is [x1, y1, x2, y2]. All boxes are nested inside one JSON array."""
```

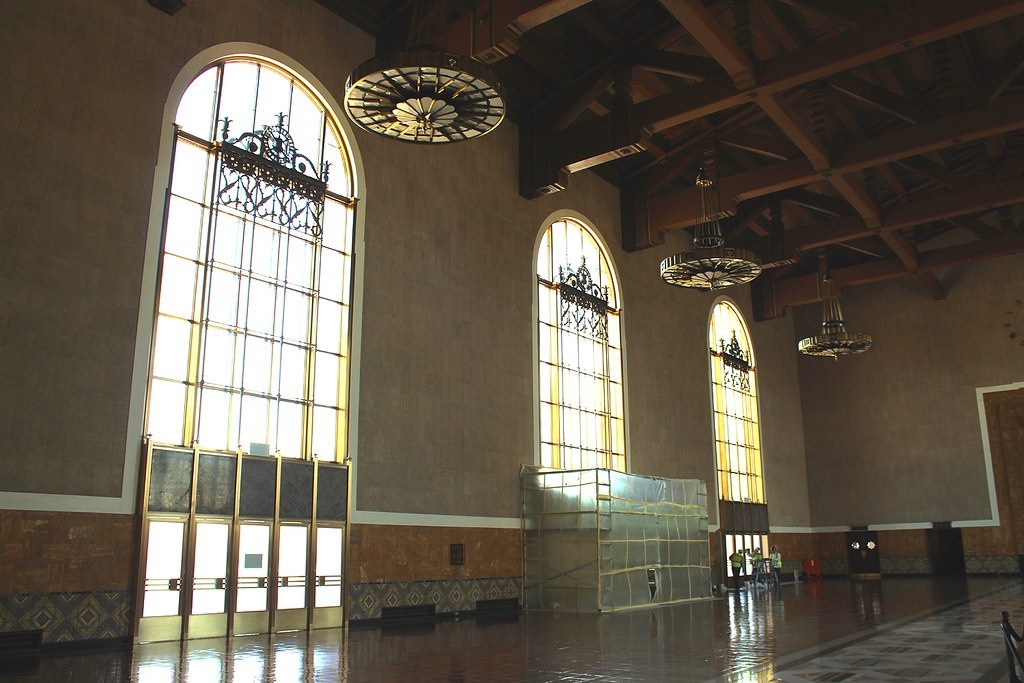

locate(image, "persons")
[[769, 545, 783, 586], [746, 547, 763, 574], [729, 549, 745, 595]]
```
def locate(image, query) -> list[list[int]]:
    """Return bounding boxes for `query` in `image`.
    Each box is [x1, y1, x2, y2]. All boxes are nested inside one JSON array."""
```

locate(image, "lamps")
[[798, 246, 871, 363], [659, 117, 762, 293], [343, 0, 507, 145]]
